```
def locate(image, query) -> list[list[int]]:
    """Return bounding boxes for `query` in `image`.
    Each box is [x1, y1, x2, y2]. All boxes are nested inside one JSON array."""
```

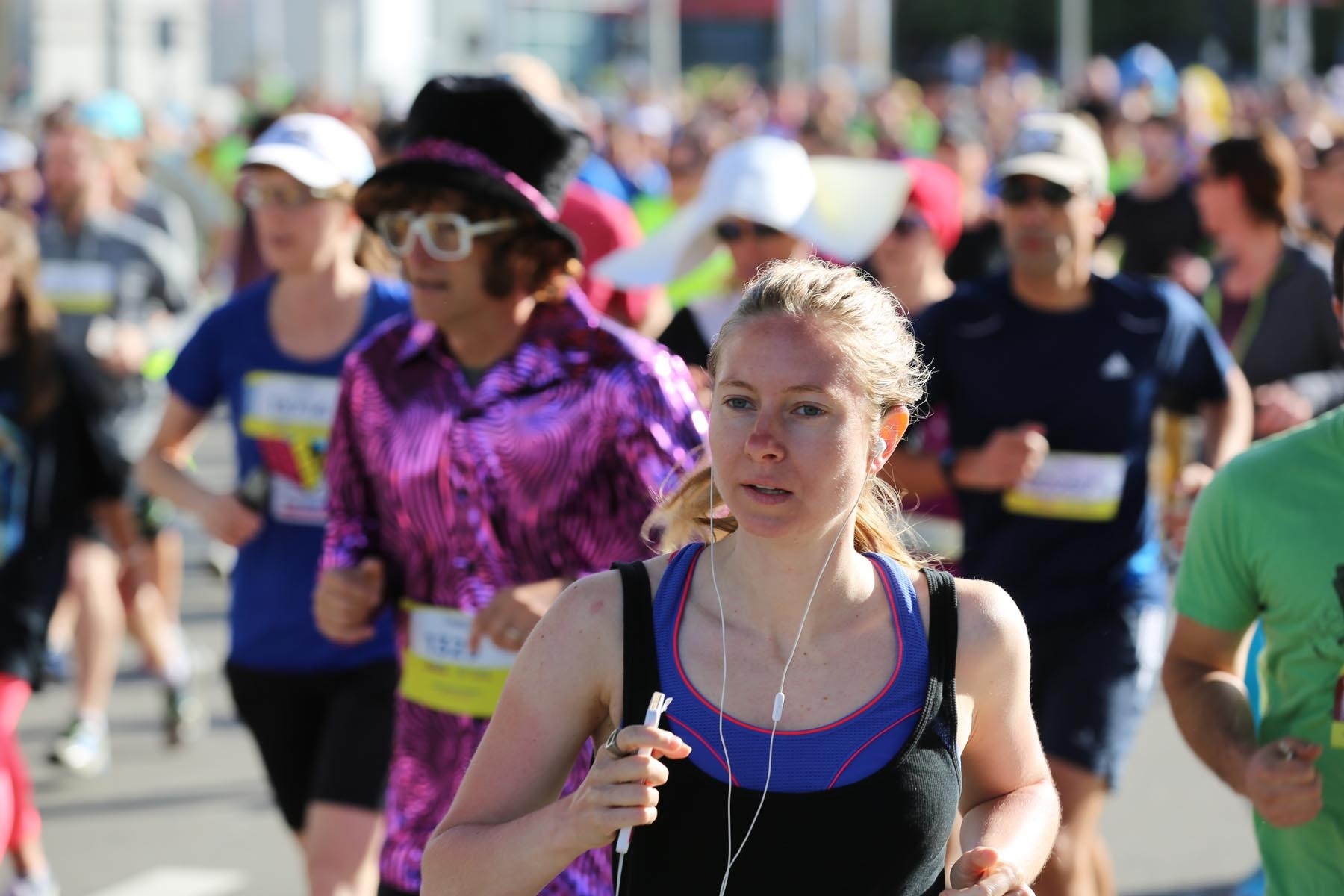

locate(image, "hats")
[[352, 75, 584, 270], [898, 158, 962, 256], [589, 137, 907, 289], [80, 90, 141, 140], [983, 112, 1108, 200], [238, 111, 374, 190]]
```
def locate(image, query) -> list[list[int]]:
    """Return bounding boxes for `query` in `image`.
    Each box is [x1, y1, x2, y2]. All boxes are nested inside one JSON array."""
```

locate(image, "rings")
[[605, 729, 629, 757]]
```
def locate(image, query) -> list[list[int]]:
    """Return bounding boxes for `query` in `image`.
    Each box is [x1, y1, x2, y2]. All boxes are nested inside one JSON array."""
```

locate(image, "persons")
[[313, 76, 710, 896], [1163, 226, 1344, 896], [418, 260, 1063, 896], [0, 39, 1344, 896]]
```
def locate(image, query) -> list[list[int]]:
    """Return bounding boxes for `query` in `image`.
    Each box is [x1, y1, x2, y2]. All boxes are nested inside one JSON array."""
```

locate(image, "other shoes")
[[47, 719, 116, 776], [166, 673, 215, 747], [17, 871, 60, 896]]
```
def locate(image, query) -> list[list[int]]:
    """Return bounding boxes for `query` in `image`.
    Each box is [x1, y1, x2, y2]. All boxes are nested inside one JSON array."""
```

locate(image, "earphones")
[[871, 436, 886, 456]]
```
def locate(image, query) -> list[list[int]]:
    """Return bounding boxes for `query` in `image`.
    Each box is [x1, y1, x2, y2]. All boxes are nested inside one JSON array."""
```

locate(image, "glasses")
[[715, 222, 775, 243], [1002, 180, 1070, 207], [238, 178, 324, 213], [895, 213, 928, 237], [376, 206, 524, 262]]
